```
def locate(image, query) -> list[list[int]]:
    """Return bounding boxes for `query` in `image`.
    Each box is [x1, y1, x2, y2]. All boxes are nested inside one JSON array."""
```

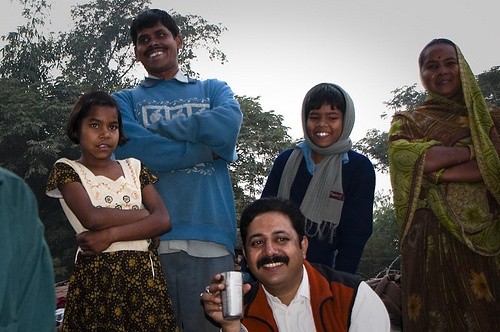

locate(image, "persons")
[[0, 168, 56, 332], [46, 91, 178, 332], [200, 198, 391, 332], [388, 38, 500, 332], [260, 82, 376, 275], [110, 9, 243, 332]]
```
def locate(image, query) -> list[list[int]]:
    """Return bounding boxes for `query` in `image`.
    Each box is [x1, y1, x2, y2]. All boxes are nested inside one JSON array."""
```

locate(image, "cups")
[[219, 271, 244, 320]]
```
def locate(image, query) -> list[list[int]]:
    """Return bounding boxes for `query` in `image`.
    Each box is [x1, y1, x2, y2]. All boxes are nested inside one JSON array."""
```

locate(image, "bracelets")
[[466, 143, 475, 160], [431, 168, 445, 184]]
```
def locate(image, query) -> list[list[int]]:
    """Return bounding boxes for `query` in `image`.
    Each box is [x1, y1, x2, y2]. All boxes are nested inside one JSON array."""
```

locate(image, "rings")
[[205, 286, 213, 294], [199, 292, 204, 297]]
